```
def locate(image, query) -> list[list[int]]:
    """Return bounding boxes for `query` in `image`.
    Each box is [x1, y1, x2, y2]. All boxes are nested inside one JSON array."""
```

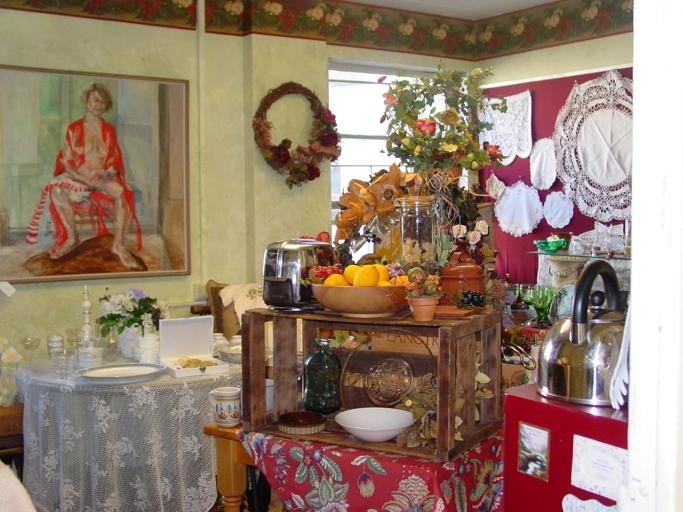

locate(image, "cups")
[[519, 283, 534, 304], [208, 387, 241, 428]]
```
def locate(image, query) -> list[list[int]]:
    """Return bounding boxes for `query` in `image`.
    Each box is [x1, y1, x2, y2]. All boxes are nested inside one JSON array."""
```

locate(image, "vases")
[[405, 296, 441, 322], [428, 166, 462, 189], [114, 324, 140, 358]]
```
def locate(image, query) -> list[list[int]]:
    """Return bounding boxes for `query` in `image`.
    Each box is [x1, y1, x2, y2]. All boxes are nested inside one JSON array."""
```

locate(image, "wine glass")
[[532, 286, 552, 322], [66, 329, 82, 369], [21, 336, 40, 371]]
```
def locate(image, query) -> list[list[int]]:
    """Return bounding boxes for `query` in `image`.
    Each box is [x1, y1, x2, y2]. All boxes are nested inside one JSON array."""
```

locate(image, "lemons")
[[323, 264, 408, 286]]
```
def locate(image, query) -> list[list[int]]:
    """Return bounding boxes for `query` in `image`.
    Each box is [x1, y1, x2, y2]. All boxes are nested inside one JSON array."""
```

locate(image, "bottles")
[[302, 338, 341, 415], [46, 335, 65, 375]]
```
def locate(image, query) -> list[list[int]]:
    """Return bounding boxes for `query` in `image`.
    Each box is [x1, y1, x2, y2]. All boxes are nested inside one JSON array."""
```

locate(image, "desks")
[[528, 251, 631, 292], [203, 408, 504, 511], [476, 203, 494, 249], [504, 383, 628, 512]]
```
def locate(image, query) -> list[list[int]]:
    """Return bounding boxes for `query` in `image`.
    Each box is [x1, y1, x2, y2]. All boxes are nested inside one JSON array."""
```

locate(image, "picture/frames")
[[0, 64, 191, 284]]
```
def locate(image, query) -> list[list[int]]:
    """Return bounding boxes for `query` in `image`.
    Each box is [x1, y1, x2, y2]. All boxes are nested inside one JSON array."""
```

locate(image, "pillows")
[[206, 280, 302, 351]]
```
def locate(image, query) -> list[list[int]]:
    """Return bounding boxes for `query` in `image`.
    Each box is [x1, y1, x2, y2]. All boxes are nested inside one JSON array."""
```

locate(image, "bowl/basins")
[[334, 407, 417, 442], [533, 240, 567, 254], [311, 283, 409, 318]]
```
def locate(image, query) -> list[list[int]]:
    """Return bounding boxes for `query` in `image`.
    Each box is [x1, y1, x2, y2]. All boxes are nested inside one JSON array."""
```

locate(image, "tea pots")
[[535, 259, 627, 406]]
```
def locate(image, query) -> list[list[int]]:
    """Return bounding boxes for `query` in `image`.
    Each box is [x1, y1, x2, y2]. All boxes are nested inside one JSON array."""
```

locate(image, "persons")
[[46, 82, 139, 268]]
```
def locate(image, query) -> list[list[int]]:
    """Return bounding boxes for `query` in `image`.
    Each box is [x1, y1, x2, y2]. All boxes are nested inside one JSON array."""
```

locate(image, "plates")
[[81, 363, 166, 385]]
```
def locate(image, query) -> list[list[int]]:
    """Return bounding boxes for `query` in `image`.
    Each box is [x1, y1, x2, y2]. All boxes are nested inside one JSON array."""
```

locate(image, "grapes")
[[308, 266, 344, 282]]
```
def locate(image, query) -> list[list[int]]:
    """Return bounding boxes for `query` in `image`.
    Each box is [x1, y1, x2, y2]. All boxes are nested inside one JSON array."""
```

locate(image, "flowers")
[[95, 289, 171, 337], [404, 270, 445, 298], [253, 83, 342, 190], [378, 58, 509, 170]]
